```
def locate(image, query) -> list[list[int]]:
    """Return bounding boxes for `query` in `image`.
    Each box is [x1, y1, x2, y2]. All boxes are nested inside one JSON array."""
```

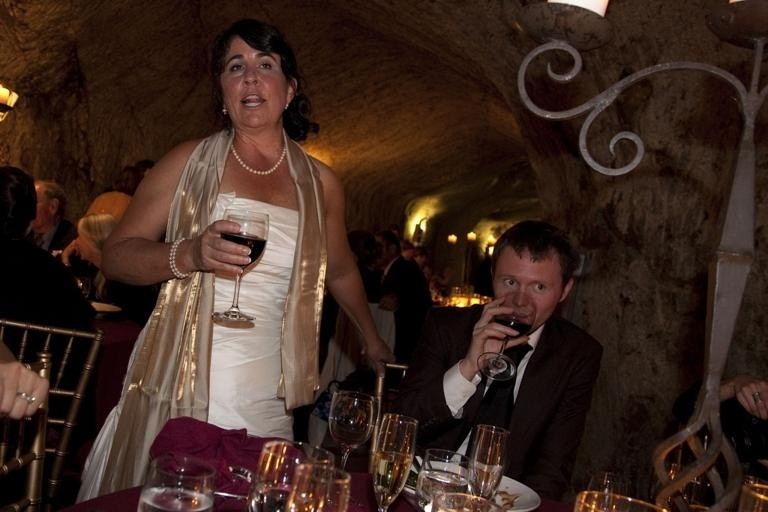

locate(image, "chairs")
[[308, 303, 395, 448], [0, 319, 104, 512], [0, 353, 52, 512], [366, 363, 406, 474]]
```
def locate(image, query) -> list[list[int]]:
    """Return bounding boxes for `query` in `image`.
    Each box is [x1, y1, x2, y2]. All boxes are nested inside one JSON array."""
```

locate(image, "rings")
[[750, 393, 761, 402], [26, 396, 36, 401], [18, 391, 28, 398]]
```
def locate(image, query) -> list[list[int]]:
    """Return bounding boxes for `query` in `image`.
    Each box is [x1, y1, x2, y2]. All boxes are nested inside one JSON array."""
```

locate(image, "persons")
[[397, 213, 609, 497], [1, 166, 131, 377], [319, 229, 435, 377], [0, 336, 50, 419], [672, 361, 767, 460], [77, 13, 392, 503]]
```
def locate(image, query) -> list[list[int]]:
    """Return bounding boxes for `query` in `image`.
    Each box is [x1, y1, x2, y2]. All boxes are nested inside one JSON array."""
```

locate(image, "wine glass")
[[368, 412, 419, 512], [476, 291, 537, 382], [327, 390, 377, 471], [212, 209, 270, 323]]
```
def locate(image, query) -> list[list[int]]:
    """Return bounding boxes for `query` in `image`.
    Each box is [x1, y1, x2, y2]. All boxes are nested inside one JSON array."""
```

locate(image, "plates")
[[402, 454, 542, 512]]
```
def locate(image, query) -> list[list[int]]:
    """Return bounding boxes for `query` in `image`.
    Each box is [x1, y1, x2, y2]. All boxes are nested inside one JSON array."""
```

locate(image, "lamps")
[[0, 82, 19, 122]]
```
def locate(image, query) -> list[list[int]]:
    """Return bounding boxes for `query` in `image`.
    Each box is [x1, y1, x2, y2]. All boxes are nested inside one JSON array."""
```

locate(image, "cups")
[[586, 470, 632, 497], [468, 423, 511, 500], [246, 439, 306, 512], [573, 489, 670, 512], [135, 450, 218, 512], [737, 482, 768, 512], [430, 492, 507, 511], [285, 463, 352, 512], [291, 441, 335, 471], [414, 447, 474, 504]]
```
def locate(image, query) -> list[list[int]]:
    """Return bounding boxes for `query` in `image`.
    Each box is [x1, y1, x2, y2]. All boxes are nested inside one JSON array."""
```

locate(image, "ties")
[[463, 342, 534, 466]]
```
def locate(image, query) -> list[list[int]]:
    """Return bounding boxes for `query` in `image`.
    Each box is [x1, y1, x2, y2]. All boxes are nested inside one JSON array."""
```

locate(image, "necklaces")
[[228, 139, 294, 177]]
